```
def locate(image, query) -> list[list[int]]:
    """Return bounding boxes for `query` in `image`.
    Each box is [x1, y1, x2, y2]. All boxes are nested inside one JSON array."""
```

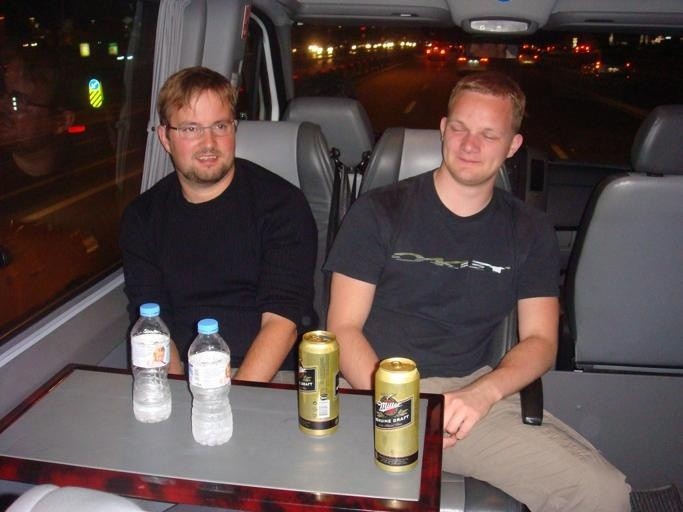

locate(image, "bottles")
[[128, 302, 173, 424], [188, 318, 233, 446]]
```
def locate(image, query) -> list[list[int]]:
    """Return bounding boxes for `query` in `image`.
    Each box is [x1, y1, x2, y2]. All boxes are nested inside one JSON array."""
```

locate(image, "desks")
[[0, 363, 447, 511]]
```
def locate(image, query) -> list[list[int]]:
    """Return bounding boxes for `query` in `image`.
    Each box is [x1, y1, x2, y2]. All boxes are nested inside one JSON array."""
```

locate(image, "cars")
[[307, 40, 416, 60], [422, 39, 630, 84]]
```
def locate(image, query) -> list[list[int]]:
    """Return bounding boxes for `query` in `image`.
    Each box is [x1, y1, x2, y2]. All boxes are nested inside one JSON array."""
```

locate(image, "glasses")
[[162, 120, 237, 139]]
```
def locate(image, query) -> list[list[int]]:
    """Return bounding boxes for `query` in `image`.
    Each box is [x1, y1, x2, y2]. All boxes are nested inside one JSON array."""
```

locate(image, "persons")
[[322, 64, 635, 511], [1, 49, 89, 203], [117, 66, 319, 385]]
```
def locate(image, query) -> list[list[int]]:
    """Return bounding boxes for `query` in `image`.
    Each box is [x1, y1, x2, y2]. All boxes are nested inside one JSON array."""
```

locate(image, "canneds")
[[373, 356, 420, 473], [297, 330, 340, 437]]
